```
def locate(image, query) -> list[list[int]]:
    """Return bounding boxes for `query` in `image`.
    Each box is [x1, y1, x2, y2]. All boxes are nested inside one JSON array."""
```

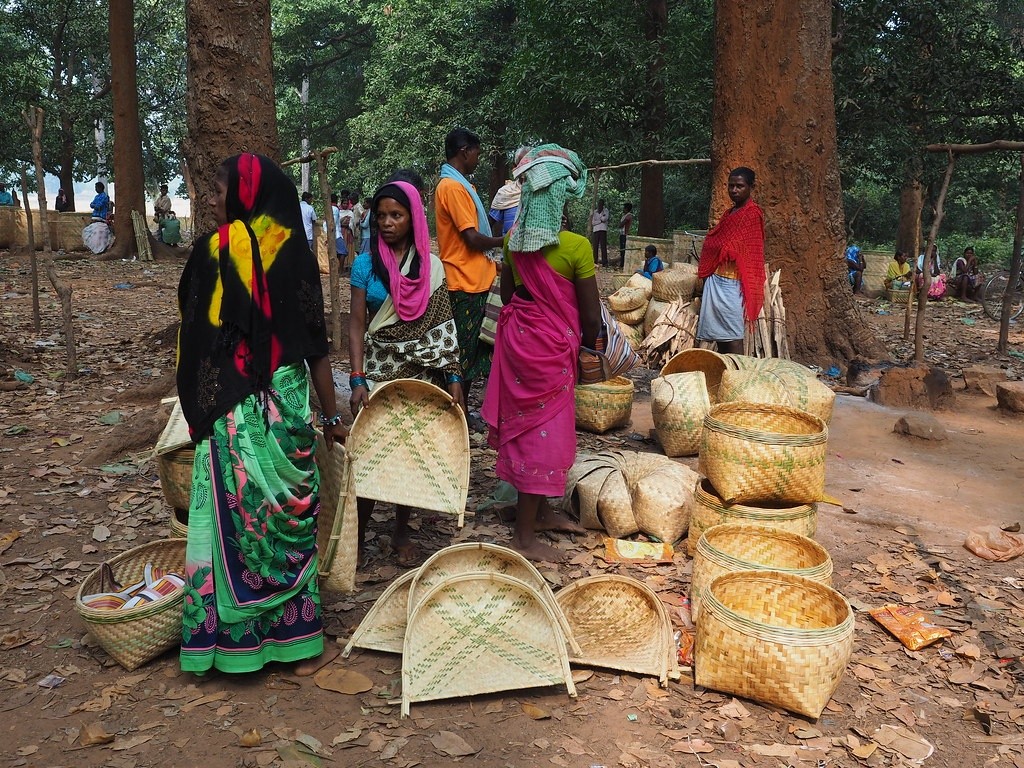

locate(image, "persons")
[[844, 239, 867, 296], [614, 203, 632, 269], [950, 247, 981, 304], [698, 166, 766, 355], [0, 182, 14, 205], [349, 185, 469, 578], [430, 127, 505, 432], [328, 187, 372, 276], [90, 182, 108, 222], [55, 187, 71, 212], [177, 151, 350, 674], [884, 249, 913, 298], [913, 241, 950, 302], [483, 142, 605, 566], [155, 184, 179, 247], [635, 244, 664, 282], [590, 198, 609, 268], [299, 191, 317, 252]]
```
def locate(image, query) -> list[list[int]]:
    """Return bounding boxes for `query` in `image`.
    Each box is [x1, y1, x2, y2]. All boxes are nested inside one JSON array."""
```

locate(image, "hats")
[[159, 185, 171, 194]]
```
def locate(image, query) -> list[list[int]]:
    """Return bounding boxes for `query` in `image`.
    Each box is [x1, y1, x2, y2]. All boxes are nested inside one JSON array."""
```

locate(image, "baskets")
[[76, 537, 189, 673], [159, 447, 195, 512], [608, 261, 703, 350], [886, 287, 910, 303], [555, 348, 837, 551], [687, 476, 817, 556], [696, 570, 854, 718], [170, 507, 188, 537], [573, 376, 634, 435], [691, 522, 834, 623]]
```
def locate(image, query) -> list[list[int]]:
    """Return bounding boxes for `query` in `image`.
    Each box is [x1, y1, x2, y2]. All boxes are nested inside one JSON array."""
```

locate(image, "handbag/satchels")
[[81, 562, 186, 612], [578, 297, 643, 384]]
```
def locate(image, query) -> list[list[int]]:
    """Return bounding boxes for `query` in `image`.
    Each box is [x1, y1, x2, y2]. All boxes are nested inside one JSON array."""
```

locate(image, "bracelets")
[[973, 267, 978, 269], [860, 262, 863, 264], [447, 374, 462, 383], [349, 370, 370, 392], [319, 411, 344, 425]]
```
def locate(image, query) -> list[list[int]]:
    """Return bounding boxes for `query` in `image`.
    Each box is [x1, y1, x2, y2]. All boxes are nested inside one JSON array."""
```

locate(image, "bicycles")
[[982, 246, 1024, 322], [682, 228, 708, 265]]
